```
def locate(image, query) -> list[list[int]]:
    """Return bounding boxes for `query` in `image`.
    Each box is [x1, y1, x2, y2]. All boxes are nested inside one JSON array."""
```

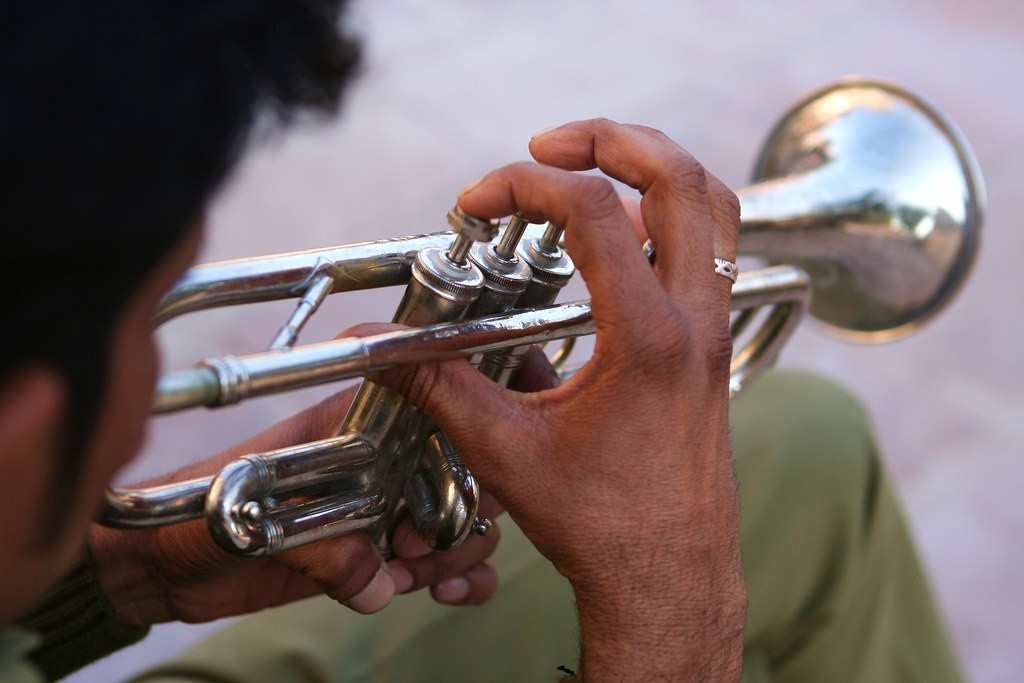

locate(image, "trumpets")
[[93, 77, 989, 578]]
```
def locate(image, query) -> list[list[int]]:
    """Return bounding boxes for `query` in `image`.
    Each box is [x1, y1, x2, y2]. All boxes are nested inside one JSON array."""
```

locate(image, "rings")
[[714, 258, 740, 284]]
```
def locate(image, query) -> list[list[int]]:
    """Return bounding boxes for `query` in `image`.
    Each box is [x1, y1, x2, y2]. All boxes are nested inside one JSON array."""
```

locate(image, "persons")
[[0, 0, 966, 683]]
